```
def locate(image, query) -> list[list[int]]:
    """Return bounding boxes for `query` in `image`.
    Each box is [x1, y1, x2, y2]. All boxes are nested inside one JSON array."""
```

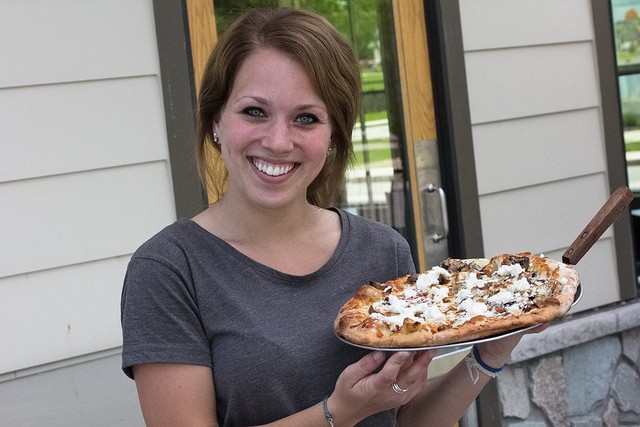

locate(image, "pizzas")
[[334, 251, 580, 349]]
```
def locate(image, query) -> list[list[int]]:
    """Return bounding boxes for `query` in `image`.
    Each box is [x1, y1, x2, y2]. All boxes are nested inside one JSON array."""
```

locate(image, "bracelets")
[[323, 393, 338, 426], [465, 344, 504, 383]]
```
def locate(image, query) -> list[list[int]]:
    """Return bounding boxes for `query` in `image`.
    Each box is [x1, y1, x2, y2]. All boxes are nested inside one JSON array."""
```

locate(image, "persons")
[[120, 6, 553, 426]]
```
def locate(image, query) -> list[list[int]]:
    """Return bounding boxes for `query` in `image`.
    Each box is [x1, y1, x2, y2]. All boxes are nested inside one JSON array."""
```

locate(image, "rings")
[[391, 379, 409, 395]]
